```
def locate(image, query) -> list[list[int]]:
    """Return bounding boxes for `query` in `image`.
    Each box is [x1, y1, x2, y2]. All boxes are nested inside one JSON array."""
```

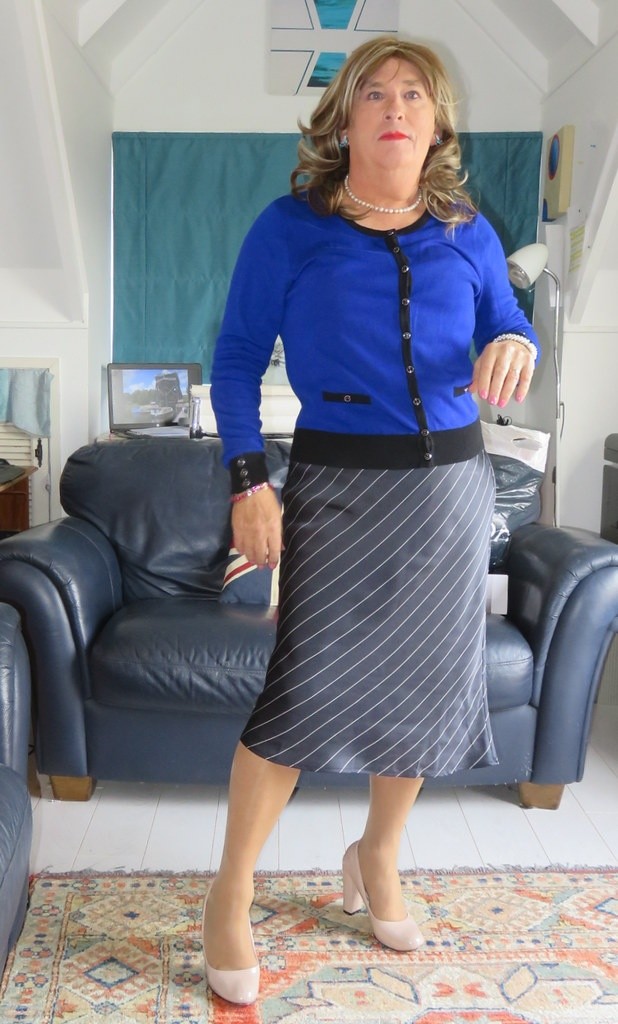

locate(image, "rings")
[[509, 368, 522, 376]]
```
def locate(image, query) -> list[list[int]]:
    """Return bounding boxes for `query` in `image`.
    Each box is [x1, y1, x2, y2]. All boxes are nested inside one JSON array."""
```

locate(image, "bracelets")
[[230, 481, 271, 504], [492, 333, 537, 361]]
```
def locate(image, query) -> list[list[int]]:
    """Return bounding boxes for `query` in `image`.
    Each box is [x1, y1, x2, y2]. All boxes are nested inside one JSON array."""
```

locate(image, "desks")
[[1, 464, 37, 541]]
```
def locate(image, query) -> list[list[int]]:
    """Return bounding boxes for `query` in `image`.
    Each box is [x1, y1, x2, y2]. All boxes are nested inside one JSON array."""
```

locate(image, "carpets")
[[0, 864, 617, 1022]]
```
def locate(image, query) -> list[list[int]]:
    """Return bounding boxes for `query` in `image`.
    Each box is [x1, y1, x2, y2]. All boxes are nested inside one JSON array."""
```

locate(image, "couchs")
[[1, 599, 37, 993], [1, 433, 616, 809]]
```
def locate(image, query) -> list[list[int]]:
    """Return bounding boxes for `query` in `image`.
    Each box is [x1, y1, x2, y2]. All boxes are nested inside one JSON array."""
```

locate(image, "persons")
[[201, 36, 544, 1006]]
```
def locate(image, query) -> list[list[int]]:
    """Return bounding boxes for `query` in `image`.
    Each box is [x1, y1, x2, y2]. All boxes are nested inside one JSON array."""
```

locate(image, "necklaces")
[[343, 173, 423, 213]]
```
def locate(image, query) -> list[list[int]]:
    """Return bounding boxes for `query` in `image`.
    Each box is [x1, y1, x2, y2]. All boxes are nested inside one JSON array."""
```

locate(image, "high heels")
[[342, 838, 425, 952], [201, 877, 261, 1005]]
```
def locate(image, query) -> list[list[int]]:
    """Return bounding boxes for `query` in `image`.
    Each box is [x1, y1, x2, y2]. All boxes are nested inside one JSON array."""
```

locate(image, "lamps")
[[503, 240, 568, 422]]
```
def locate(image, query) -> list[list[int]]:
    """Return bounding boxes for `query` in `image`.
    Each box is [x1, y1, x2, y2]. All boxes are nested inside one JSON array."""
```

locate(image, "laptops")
[[107, 363, 202, 438]]
